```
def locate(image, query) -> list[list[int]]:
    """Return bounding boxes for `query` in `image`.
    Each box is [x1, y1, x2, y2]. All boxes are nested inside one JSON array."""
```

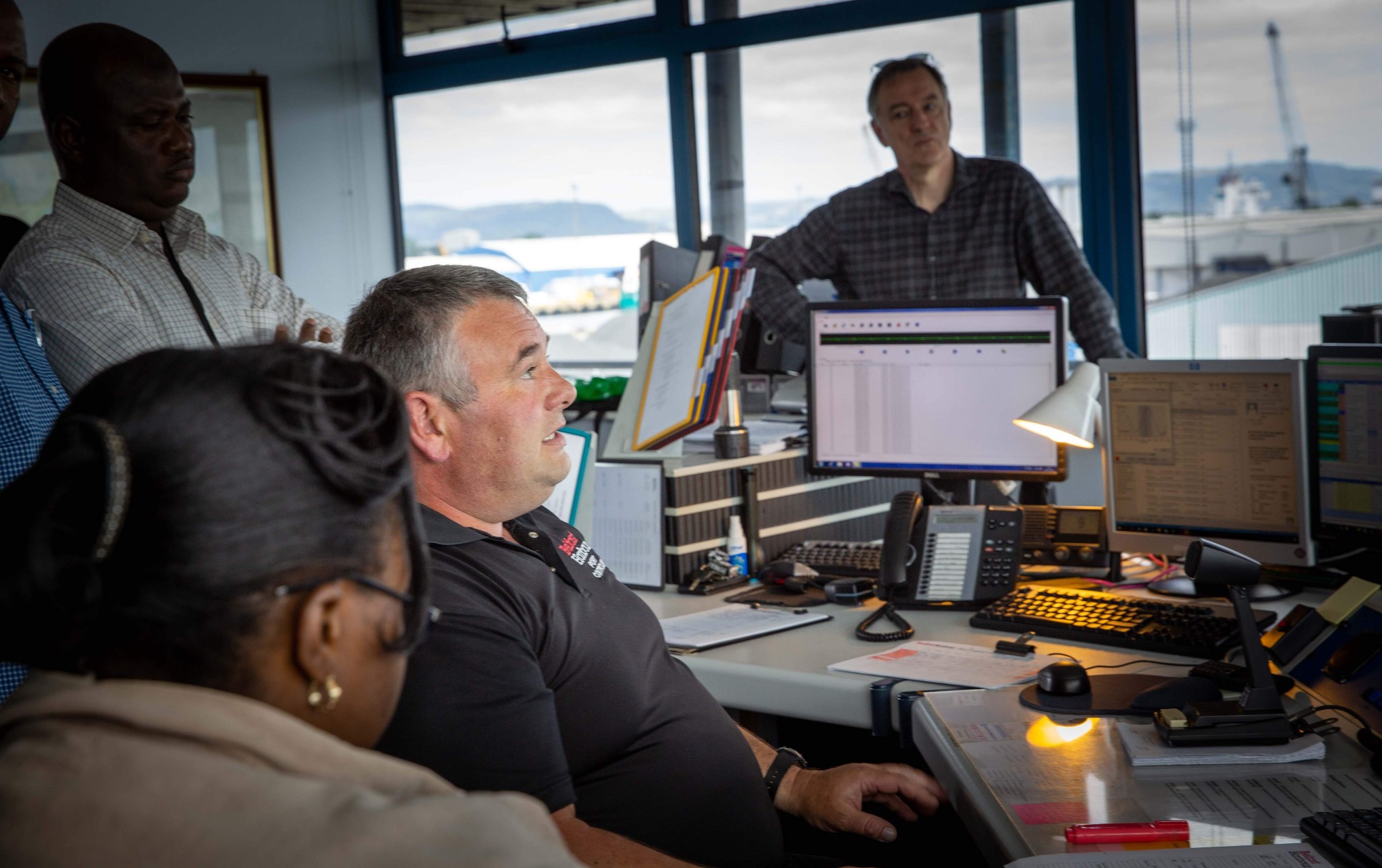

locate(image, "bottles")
[[726, 515, 747, 575]]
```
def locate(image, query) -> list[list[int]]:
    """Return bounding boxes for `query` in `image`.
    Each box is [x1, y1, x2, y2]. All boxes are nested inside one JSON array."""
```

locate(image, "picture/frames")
[[0, 72, 286, 283]]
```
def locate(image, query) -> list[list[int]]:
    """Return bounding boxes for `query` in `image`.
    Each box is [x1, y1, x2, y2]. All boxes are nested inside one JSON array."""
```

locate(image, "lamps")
[[1014, 362, 1149, 588]]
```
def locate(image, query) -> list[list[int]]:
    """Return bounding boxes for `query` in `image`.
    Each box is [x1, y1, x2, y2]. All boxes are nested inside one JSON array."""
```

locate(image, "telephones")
[[877, 489, 1022, 612]]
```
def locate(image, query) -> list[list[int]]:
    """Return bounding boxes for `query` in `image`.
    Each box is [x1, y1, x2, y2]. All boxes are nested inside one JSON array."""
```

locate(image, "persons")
[[745, 52, 1130, 370], [345, 267, 950, 868], [2, 3, 76, 486], [0, 344, 597, 868], [0, 21, 345, 396]]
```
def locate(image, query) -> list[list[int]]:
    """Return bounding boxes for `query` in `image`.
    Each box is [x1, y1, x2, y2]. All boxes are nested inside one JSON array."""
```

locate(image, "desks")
[[623, 578, 1382, 868]]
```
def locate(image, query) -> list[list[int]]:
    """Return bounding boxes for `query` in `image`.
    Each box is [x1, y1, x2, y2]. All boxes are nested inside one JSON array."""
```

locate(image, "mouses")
[[1038, 660, 1091, 696]]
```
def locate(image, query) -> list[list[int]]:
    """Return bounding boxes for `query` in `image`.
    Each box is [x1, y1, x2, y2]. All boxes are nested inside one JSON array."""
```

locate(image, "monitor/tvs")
[[1303, 341, 1382, 535], [1092, 360, 1318, 568], [806, 299, 1065, 485]]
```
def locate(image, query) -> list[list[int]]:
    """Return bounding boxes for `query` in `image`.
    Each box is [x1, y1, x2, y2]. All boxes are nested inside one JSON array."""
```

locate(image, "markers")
[[1065, 818, 1189, 843]]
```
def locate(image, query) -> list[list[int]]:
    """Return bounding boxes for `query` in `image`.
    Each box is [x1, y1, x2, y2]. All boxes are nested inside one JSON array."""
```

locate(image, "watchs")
[[763, 742, 806, 807]]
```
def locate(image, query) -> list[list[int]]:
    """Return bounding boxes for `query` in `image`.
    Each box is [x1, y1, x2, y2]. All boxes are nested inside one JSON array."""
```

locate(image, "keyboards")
[[1299, 805, 1382, 868], [967, 586, 1275, 662], [759, 542, 887, 579]]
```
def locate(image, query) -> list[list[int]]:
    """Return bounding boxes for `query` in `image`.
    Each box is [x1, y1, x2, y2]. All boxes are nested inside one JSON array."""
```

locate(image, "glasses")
[[865, 52, 937, 81], [276, 571, 443, 656]]
